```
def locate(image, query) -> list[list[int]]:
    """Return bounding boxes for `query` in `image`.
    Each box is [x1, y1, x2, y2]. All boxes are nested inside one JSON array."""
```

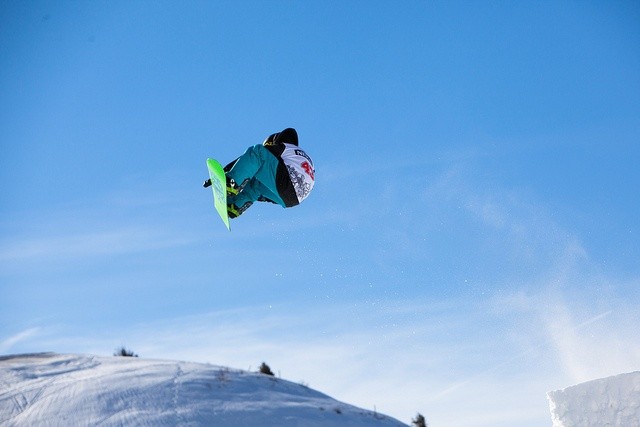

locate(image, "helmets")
[[263, 133, 280, 145]]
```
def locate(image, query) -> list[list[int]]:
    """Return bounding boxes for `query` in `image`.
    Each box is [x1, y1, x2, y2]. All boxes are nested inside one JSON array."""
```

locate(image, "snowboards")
[[206, 157, 231, 231]]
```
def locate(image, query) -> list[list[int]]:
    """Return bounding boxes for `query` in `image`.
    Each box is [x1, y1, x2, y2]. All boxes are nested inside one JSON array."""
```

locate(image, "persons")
[[202, 127, 315, 219]]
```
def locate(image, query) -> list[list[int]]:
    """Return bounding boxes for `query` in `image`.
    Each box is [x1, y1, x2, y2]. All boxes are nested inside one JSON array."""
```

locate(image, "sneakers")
[[227, 203, 242, 219], [226, 177, 240, 195]]
